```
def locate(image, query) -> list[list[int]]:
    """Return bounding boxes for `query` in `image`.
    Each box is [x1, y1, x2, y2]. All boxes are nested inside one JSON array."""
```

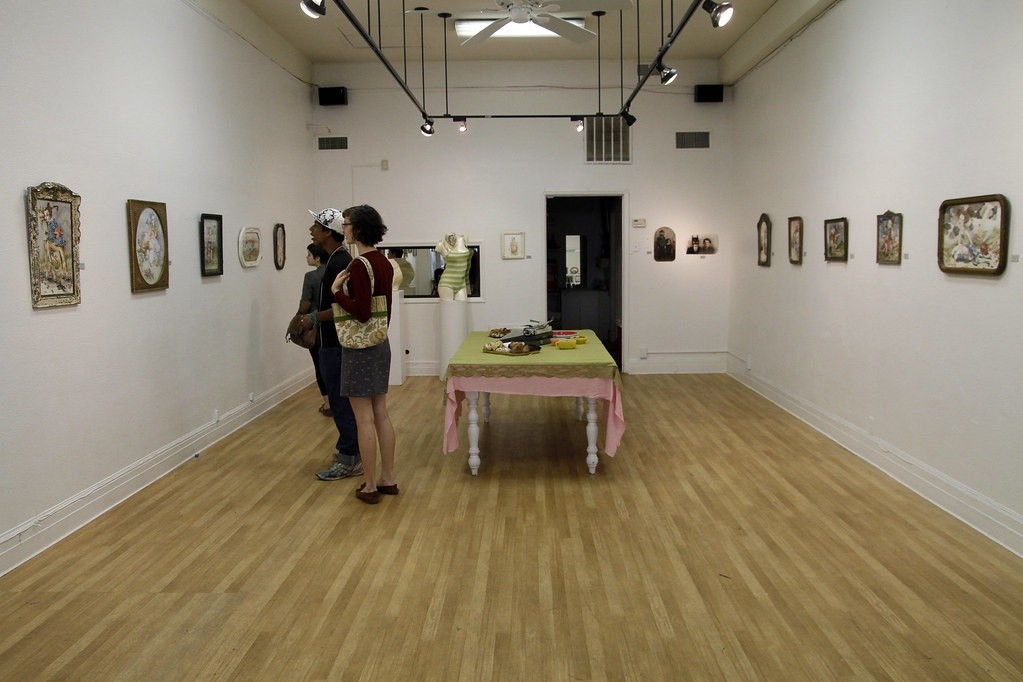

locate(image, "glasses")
[[342, 223, 354, 229]]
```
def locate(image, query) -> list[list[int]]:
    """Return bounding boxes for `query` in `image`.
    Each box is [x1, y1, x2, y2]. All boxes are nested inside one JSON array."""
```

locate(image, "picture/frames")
[[876, 210, 904, 265], [273, 223, 287, 270], [200, 213, 223, 277], [824, 217, 849, 262], [128, 199, 170, 293], [237, 226, 262, 268], [757, 212, 772, 267], [27, 180, 81, 308], [936, 193, 1011, 276], [788, 217, 804, 264], [501, 231, 525, 259]]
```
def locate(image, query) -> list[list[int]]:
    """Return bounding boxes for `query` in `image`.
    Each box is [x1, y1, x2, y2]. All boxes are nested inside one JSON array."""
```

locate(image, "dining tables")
[[441, 329, 625, 476]]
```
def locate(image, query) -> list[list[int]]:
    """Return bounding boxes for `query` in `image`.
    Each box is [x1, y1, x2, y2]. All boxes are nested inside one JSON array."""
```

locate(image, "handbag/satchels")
[[331, 255, 388, 349], [285, 312, 317, 349]]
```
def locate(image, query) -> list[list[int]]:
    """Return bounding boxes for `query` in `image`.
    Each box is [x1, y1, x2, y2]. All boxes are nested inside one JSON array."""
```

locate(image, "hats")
[[308, 208, 345, 236]]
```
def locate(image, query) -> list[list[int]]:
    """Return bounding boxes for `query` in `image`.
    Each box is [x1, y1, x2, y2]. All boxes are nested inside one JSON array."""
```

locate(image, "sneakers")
[[315, 453, 365, 481]]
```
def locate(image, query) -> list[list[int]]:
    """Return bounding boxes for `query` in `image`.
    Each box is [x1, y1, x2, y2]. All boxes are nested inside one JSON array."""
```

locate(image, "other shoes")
[[319, 403, 333, 417], [356, 488, 379, 504], [360, 481, 399, 495]]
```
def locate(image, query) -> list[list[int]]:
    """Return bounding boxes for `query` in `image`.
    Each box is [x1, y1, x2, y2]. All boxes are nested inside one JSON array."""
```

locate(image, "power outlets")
[[215, 409, 221, 422], [640, 346, 648, 359], [249, 392, 254, 401], [866, 416, 874, 434], [744, 354, 752, 369], [381, 159, 389, 171]]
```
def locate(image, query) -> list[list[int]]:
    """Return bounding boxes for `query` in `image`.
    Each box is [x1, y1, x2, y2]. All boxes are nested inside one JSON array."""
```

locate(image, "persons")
[[296, 242, 333, 416], [435, 232, 470, 300], [305, 208, 364, 481], [331, 204, 400, 505], [465, 247, 475, 294], [387, 247, 403, 259]]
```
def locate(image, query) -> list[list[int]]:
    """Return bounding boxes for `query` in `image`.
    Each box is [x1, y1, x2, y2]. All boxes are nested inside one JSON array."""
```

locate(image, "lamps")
[[299, 0, 326, 19], [620, 104, 636, 126], [420, 118, 435, 137], [570, 117, 585, 132], [702, 0, 734, 28], [454, 18, 585, 39], [453, 117, 467, 131], [656, 52, 679, 86]]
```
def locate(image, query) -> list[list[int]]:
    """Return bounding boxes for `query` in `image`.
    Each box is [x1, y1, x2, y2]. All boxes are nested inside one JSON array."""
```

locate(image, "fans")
[[402, 0, 634, 46]]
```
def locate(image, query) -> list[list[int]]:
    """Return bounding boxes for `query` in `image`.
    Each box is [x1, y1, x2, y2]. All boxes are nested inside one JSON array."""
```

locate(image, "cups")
[[555, 339, 576, 349]]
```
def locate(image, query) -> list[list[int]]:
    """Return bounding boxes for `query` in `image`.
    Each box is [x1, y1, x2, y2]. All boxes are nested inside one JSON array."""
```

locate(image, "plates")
[[552, 331, 578, 338]]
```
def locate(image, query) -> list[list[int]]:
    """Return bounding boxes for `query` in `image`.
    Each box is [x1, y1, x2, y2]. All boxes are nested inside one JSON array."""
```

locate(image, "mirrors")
[[375, 241, 485, 303]]
